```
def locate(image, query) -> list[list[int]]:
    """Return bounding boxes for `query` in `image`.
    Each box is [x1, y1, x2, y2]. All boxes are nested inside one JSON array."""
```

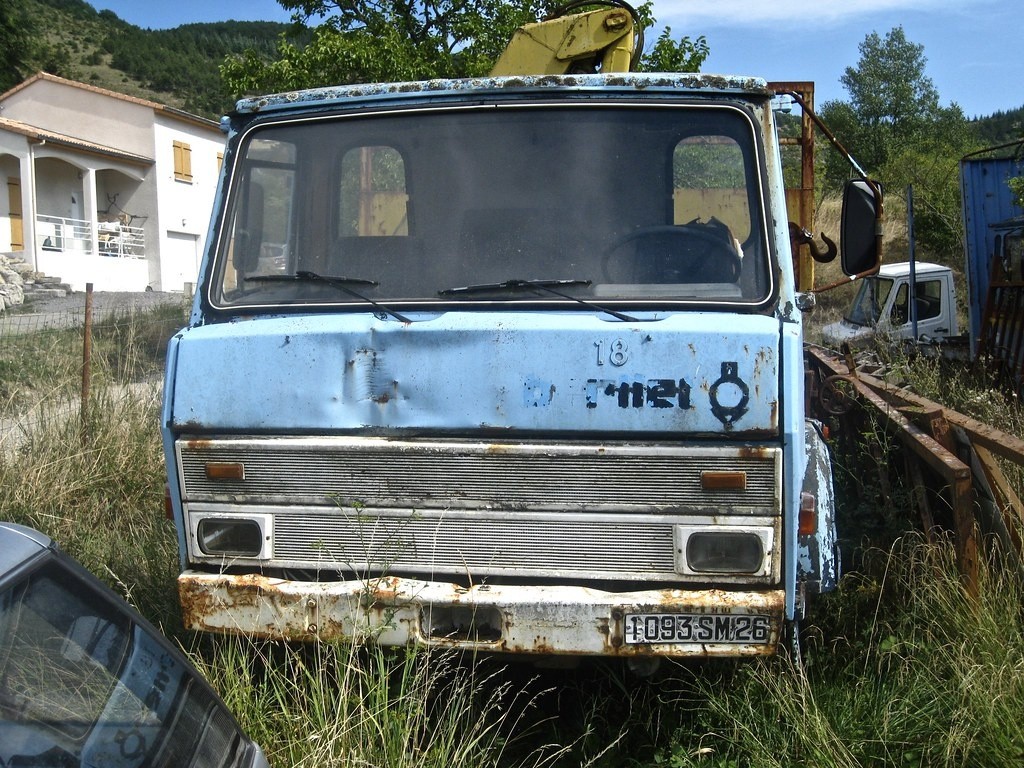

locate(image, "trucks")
[[813, 260, 965, 352]]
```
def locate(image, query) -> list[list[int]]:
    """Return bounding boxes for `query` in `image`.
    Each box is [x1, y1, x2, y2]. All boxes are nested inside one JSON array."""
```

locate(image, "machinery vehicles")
[[149, 0, 844, 671]]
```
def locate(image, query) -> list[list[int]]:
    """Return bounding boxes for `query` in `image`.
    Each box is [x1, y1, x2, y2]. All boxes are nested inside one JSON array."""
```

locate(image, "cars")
[[1, 523, 270, 768]]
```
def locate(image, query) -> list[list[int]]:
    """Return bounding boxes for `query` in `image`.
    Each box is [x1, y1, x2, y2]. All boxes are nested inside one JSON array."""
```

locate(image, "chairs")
[[98, 214, 129, 255], [634, 216, 735, 283], [328, 233, 425, 300]]
